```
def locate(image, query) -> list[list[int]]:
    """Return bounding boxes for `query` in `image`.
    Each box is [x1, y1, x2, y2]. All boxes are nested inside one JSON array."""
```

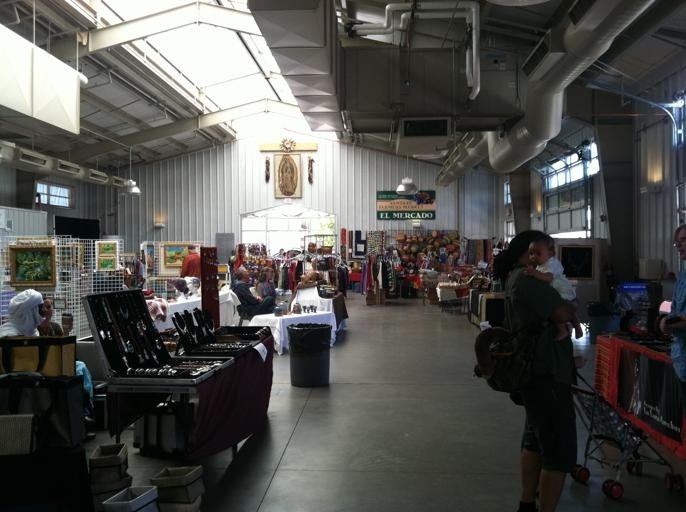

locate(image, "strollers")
[[570, 369, 684, 500]]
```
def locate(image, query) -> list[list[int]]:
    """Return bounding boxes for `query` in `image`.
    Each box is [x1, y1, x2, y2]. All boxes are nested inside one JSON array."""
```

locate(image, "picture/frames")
[[94, 240, 120, 272], [164, 243, 189, 266], [9, 243, 55, 288]]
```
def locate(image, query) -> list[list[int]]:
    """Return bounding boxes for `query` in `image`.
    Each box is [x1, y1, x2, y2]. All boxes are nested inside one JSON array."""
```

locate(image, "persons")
[[231, 264, 275, 319], [492, 229, 576, 512], [256, 267, 276, 299], [180, 244, 201, 277], [522, 236, 583, 342], [38, 298, 97, 439], [0, 288, 46, 337], [660, 224, 686, 382]]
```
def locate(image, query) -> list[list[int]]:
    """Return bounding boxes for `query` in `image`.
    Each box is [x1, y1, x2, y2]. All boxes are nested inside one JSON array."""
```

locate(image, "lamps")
[[120, 143, 142, 198], [396, 153, 419, 195]]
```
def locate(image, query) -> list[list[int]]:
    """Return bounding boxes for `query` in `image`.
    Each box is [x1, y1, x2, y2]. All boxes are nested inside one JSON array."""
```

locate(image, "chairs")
[[229, 288, 256, 328]]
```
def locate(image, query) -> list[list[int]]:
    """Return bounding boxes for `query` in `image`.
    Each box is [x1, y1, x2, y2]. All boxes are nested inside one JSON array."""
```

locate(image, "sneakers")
[[81, 415, 95, 424], [86, 432, 96, 439]]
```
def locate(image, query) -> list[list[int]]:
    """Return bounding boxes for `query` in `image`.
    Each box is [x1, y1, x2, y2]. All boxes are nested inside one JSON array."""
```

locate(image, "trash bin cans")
[[288, 323, 331, 388]]
